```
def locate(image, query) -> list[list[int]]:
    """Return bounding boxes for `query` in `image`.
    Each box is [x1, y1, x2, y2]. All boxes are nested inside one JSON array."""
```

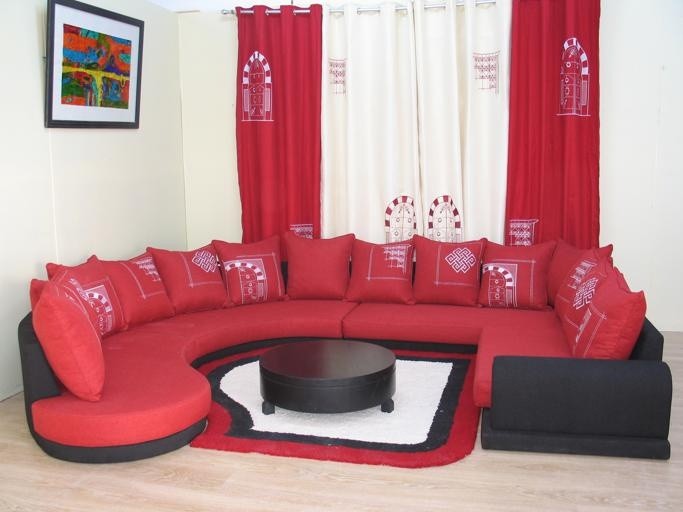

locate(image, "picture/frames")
[[44, 1, 144, 129]]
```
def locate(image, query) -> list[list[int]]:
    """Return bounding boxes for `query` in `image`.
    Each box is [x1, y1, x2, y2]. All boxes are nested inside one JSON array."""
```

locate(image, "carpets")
[[189, 348, 481, 468]]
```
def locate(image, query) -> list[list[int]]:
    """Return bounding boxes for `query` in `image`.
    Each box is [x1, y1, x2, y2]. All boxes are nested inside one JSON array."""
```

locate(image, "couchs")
[[18, 261, 673, 463]]
